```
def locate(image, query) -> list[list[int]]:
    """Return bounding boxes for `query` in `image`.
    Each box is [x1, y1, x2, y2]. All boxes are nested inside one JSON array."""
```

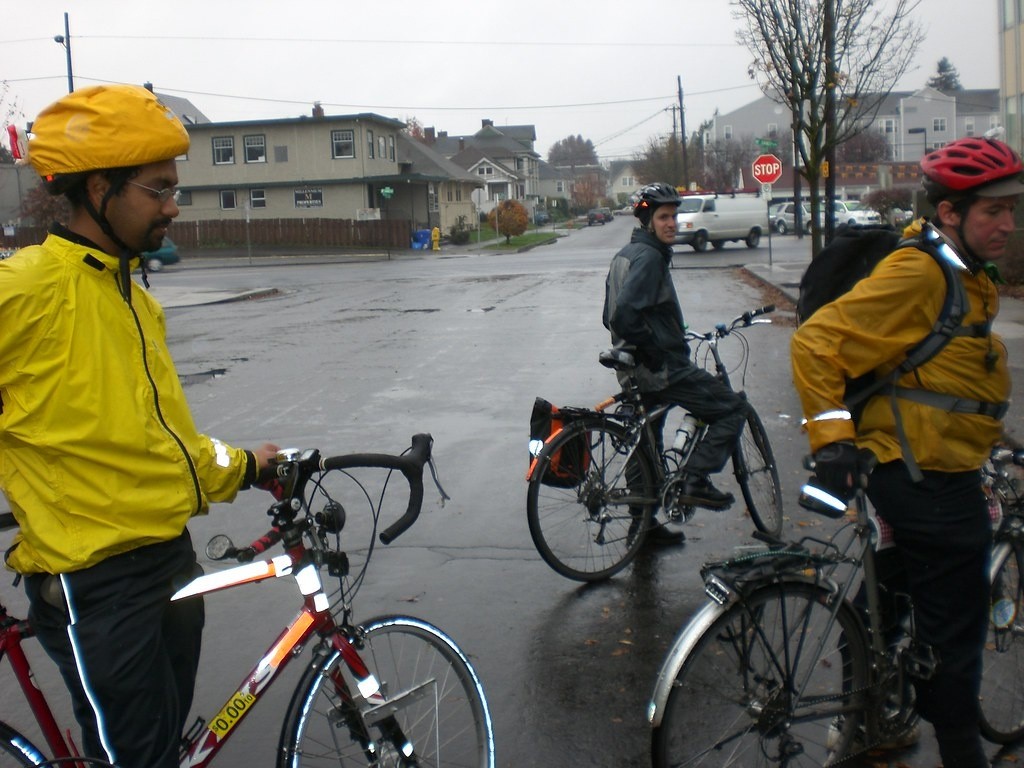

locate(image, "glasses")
[[127, 179, 181, 203]]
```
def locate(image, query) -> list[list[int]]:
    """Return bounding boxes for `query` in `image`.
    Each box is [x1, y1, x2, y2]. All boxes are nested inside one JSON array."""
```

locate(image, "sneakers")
[[828, 707, 922, 752], [678, 476, 736, 509], [626, 516, 686, 546]]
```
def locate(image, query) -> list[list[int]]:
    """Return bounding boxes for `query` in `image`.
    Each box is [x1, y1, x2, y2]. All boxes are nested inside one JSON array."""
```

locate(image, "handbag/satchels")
[[530, 396, 592, 489]]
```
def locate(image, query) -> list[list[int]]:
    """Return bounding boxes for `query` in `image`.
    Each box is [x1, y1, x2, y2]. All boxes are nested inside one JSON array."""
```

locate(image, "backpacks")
[[796, 222, 970, 430]]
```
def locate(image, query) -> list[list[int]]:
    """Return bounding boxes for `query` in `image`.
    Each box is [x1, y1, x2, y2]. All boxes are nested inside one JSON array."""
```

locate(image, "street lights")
[[52, 11, 74, 95], [908, 127, 927, 156]]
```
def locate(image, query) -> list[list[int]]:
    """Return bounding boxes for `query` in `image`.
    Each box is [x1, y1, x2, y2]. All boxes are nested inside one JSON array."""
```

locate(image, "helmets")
[[28, 84, 190, 195], [630, 182, 682, 216], [920, 137, 1024, 203]]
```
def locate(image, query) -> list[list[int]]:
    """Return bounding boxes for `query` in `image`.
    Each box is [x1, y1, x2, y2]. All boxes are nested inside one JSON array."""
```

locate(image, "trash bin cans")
[[412, 229, 433, 250], [432, 226, 440, 250]]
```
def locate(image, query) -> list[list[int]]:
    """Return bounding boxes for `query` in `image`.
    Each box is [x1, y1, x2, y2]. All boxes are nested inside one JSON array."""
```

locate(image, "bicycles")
[[639, 436, 1024, 768], [1, 431, 497, 768], [522, 302, 785, 583]]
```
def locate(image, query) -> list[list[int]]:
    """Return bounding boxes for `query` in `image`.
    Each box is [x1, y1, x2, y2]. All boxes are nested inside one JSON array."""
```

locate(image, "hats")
[[972, 176, 1024, 197]]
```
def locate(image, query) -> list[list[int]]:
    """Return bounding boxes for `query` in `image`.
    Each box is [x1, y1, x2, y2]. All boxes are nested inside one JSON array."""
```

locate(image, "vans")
[[673, 191, 769, 253]]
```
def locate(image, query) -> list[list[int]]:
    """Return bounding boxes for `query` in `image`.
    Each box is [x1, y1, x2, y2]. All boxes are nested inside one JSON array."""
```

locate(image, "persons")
[[792, 136, 1024, 768], [602, 182, 748, 546], [0, 84, 288, 768]]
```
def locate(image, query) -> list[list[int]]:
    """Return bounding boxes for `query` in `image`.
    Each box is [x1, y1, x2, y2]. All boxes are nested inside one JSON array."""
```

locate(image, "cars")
[[768, 198, 886, 236], [136, 235, 181, 272], [587, 206, 634, 226]]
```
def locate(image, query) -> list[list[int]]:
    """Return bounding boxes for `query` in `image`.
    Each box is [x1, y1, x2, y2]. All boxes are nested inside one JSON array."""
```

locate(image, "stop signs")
[[751, 154, 783, 184]]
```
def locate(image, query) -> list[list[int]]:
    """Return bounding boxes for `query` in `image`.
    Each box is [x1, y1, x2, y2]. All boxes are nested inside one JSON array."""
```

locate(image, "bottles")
[[672, 412, 697, 454]]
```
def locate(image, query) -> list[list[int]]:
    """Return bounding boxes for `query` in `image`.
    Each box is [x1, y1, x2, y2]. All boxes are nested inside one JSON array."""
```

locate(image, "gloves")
[[812, 440, 870, 501], [640, 344, 664, 373]]
